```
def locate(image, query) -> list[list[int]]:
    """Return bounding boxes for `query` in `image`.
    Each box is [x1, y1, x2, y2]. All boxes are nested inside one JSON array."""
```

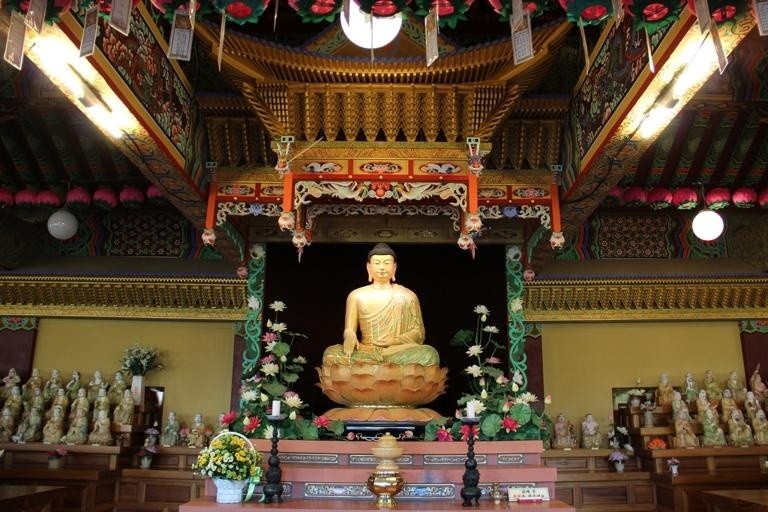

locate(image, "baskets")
[[208, 431, 257, 502]]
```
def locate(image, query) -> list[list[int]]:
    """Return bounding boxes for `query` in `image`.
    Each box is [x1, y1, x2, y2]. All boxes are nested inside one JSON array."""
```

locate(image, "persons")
[[1, 367, 230, 448], [540, 362, 768, 450], [314, 242, 440, 368]]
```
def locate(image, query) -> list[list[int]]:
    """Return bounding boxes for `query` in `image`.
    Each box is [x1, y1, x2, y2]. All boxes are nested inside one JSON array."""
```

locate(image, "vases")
[[208, 475, 250, 504], [127, 375, 147, 415]]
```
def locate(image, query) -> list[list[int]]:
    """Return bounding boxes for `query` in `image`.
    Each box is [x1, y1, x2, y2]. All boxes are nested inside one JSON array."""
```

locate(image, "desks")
[[0, 482, 67, 511], [175, 497, 578, 512], [701, 487, 767, 512]]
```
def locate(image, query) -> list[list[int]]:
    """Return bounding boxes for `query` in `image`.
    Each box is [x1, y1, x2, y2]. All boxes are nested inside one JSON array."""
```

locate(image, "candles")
[[270, 398, 281, 416], [465, 400, 477, 419]]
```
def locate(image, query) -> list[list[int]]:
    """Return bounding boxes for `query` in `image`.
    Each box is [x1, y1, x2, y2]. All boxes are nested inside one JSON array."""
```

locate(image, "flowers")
[[426, 298, 555, 449], [186, 297, 365, 440], [115, 342, 166, 380], [603, 372, 684, 481], [185, 432, 267, 480]]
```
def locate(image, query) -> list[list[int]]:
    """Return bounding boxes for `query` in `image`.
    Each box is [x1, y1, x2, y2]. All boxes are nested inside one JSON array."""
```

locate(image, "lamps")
[[41, 200, 81, 240], [631, 28, 716, 139], [52, 61, 125, 135], [336, 0, 404, 50], [689, 184, 726, 242]]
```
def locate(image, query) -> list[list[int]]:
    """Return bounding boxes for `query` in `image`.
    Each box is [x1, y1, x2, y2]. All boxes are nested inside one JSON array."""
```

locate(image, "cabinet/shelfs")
[[537, 447, 652, 483], [0, 402, 156, 486], [616, 402, 768, 486]]
